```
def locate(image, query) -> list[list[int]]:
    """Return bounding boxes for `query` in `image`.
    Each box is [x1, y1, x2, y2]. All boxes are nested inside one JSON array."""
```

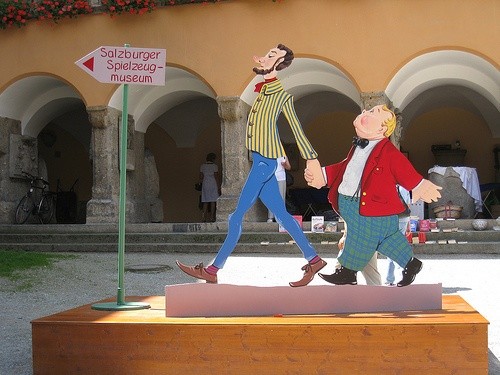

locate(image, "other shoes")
[[267, 218, 273, 222]]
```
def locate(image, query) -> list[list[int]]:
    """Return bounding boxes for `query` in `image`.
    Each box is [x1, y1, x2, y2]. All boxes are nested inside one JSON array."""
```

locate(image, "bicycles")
[[15, 171, 55, 224]]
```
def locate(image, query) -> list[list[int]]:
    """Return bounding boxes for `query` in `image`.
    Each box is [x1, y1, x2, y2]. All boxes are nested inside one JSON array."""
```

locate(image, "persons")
[[338, 183, 413, 286], [199, 152, 220, 222], [267, 157, 292, 223]]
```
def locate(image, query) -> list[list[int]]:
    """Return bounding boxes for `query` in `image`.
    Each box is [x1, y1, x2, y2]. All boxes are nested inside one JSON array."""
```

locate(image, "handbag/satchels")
[[195, 184, 202, 190]]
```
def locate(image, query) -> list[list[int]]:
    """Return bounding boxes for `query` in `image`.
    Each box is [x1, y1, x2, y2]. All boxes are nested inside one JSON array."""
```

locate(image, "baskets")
[[432, 200, 463, 220]]
